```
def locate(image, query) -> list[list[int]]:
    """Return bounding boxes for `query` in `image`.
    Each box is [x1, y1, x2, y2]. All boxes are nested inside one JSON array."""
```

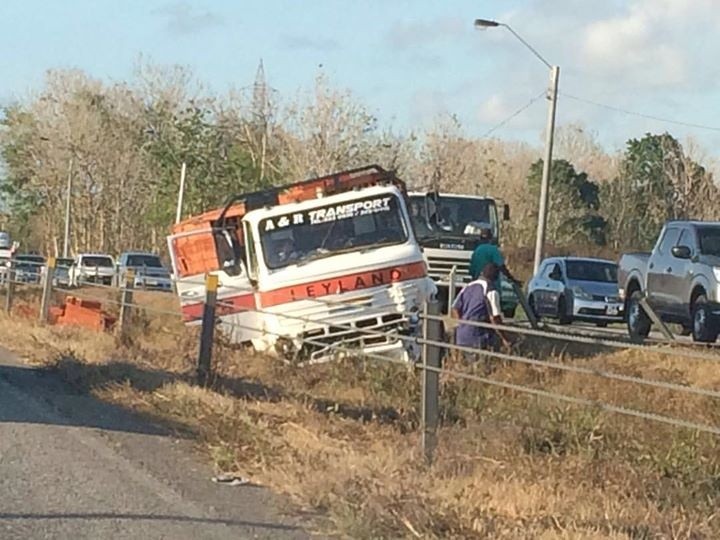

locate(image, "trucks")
[[400, 190, 520, 317], [166, 162, 433, 372]]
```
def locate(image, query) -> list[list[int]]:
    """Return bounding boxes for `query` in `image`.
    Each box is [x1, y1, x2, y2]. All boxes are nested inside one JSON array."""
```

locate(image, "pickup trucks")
[[616, 220, 720, 347]]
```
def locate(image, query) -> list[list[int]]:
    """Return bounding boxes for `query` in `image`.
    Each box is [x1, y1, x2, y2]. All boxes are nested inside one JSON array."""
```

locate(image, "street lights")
[[40, 133, 75, 258], [473, 16, 561, 276]]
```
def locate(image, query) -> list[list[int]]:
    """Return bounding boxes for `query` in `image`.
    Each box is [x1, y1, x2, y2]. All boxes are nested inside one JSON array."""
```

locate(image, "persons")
[[468, 228, 525, 310], [451, 263, 519, 379]]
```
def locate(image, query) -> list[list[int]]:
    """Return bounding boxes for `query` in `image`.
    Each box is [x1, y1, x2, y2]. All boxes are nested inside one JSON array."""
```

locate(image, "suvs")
[[115, 251, 172, 293], [67, 252, 115, 285]]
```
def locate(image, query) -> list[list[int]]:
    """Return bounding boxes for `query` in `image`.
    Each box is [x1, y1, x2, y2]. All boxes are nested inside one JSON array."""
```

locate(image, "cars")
[[9, 254, 74, 282], [526, 256, 625, 329]]
